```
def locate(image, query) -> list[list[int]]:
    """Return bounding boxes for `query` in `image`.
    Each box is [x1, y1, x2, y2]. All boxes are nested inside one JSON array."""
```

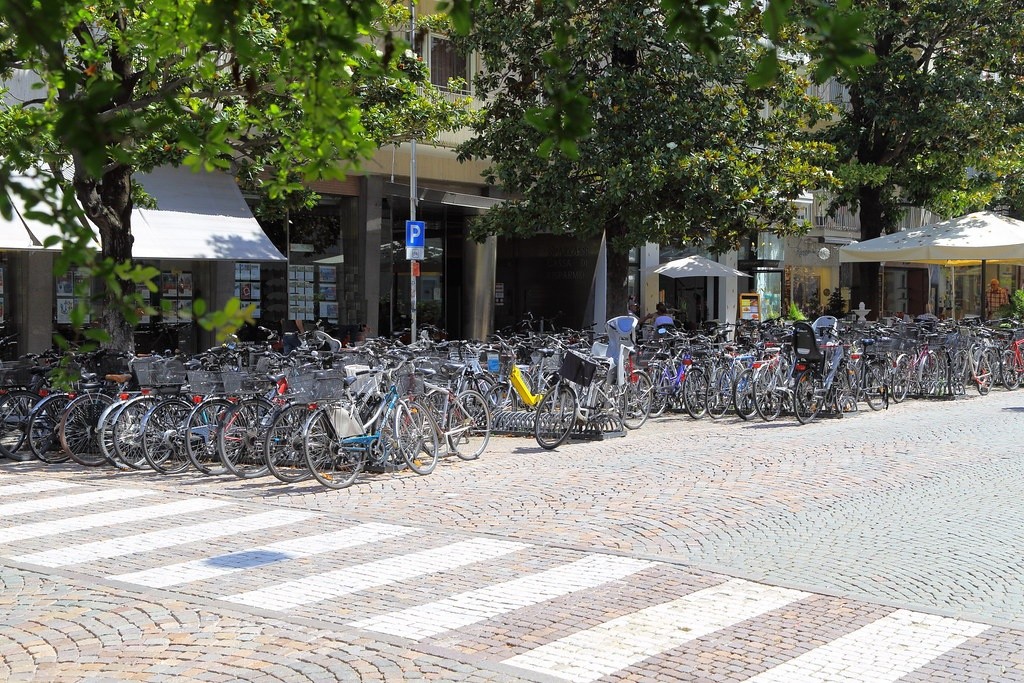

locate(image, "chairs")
[[654, 324, 676, 340]]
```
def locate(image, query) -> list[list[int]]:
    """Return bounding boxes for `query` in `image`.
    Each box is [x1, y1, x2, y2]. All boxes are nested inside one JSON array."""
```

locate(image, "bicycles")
[[0, 311, 1024, 489]]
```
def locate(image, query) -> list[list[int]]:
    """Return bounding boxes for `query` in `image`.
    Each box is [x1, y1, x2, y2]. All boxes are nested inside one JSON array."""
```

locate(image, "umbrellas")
[[839, 210, 1024, 322]]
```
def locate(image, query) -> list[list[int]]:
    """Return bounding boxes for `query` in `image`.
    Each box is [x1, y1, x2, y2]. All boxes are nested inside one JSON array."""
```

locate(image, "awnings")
[[131, 162, 287, 262], [653, 255, 754, 279], [0, 177, 104, 251]]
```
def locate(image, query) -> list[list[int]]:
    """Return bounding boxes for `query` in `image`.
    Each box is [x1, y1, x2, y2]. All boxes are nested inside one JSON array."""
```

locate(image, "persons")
[[628, 301, 676, 357], [985, 279, 1010, 319], [604, 315, 642, 364]]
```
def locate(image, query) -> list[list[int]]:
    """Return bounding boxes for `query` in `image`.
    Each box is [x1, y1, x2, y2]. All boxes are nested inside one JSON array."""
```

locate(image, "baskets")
[[349, 317, 1024, 400], [128, 355, 347, 403]]
